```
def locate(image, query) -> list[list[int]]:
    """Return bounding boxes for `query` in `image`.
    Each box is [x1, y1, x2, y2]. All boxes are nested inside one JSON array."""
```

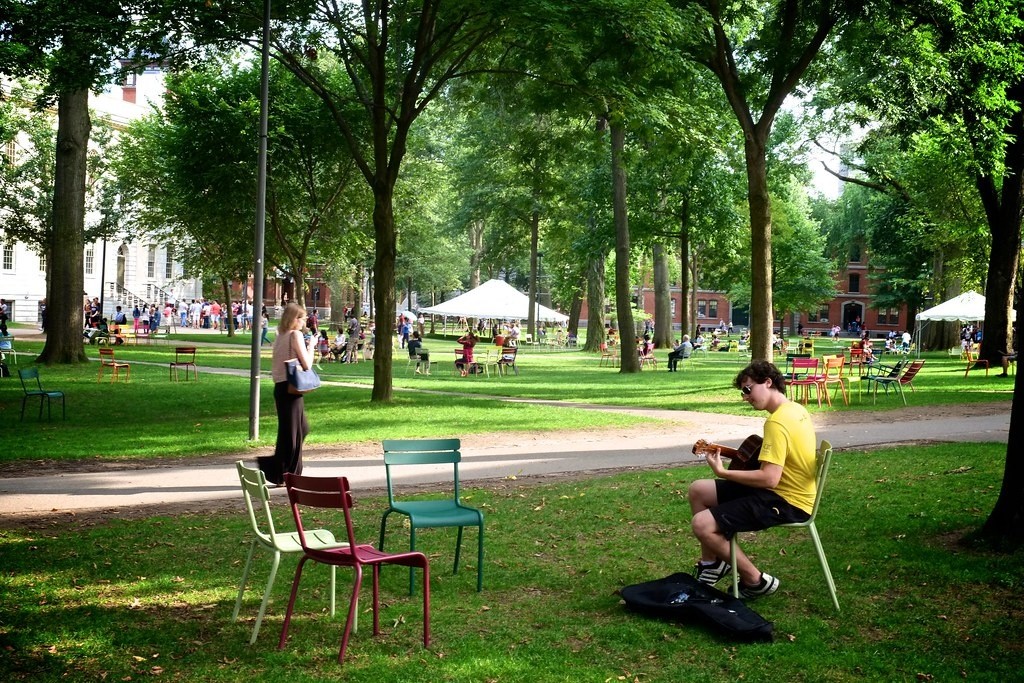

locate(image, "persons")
[[636, 318, 654, 369], [855, 315, 861, 327], [84, 297, 272, 345], [607, 328, 619, 345], [885, 330, 897, 355], [693, 324, 704, 351], [902, 330, 912, 353], [797, 323, 803, 335], [710, 318, 749, 352], [0, 298, 47, 377], [408, 330, 431, 375], [668, 334, 692, 372], [687, 358, 817, 599], [960, 325, 982, 343], [833, 324, 841, 341], [860, 330, 879, 366], [256, 303, 318, 486], [773, 330, 783, 355], [301, 305, 376, 364], [477, 319, 574, 346], [398, 312, 424, 349], [996, 351, 1017, 377], [454, 332, 477, 377]]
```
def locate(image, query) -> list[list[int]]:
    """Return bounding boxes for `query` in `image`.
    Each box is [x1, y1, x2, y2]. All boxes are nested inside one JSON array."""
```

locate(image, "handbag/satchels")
[[283, 331, 321, 394]]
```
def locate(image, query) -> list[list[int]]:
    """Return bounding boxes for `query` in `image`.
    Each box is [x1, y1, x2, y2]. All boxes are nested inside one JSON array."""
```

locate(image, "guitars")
[[692, 434, 763, 471]]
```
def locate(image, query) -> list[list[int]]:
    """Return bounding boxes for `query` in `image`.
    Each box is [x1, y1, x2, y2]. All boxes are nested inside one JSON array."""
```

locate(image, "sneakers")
[[694, 557, 732, 588], [727, 572, 779, 602]]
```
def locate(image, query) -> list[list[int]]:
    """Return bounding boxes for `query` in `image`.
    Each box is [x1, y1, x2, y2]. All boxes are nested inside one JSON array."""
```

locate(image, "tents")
[[417, 278, 569, 336], [915, 289, 1017, 359]]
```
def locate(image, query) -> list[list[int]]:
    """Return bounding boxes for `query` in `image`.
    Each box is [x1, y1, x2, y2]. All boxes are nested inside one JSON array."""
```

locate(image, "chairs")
[[377, 439, 483, 596], [96, 347, 130, 385], [452, 331, 580, 378], [948, 344, 1018, 377], [774, 339, 925, 408], [313, 332, 430, 376], [276, 472, 429, 664], [170, 346, 198, 384], [82, 318, 170, 347], [733, 441, 840, 614], [17, 367, 66, 423], [228, 459, 358, 645], [599, 333, 752, 371], [0, 336, 17, 364]]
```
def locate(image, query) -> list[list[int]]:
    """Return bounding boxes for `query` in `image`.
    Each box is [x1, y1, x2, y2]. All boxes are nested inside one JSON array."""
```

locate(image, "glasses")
[[741, 381, 757, 397]]
